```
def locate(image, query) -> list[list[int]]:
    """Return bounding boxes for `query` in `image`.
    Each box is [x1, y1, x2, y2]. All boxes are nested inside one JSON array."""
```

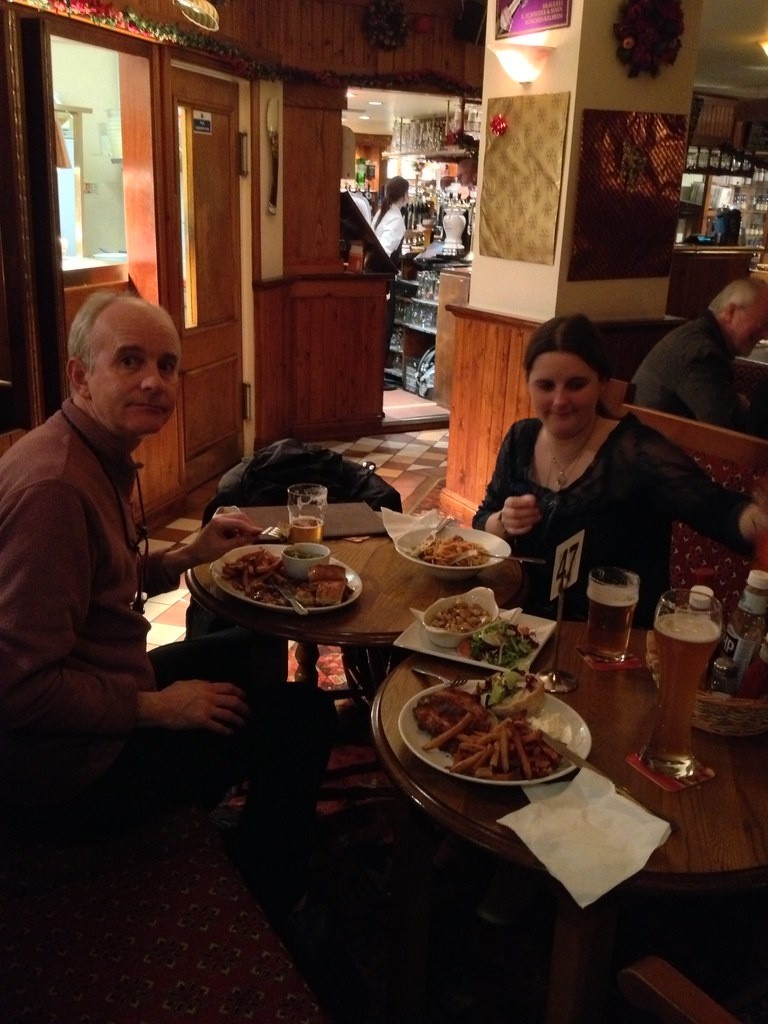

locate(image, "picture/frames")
[[496, 0, 572, 39]]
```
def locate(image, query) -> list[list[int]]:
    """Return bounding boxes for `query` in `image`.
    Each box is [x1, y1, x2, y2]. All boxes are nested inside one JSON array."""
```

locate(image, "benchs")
[[620, 354, 768, 641], [0, 801, 331, 1024]]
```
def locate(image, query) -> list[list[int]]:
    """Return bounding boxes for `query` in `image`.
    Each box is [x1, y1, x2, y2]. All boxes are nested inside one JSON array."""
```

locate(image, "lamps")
[[487, 44, 556, 86]]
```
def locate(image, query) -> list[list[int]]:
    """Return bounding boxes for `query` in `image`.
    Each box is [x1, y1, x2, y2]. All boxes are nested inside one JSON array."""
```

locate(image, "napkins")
[[497, 766, 672, 908], [379, 505, 448, 544]]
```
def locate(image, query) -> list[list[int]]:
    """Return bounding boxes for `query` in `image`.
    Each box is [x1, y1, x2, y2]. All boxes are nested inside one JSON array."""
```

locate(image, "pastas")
[[420, 540, 490, 566]]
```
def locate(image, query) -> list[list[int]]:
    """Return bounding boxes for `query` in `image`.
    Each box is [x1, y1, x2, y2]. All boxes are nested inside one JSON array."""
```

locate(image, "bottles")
[[687, 583, 714, 691], [721, 569, 768, 694], [711, 657, 737, 697], [738, 633, 768, 699]]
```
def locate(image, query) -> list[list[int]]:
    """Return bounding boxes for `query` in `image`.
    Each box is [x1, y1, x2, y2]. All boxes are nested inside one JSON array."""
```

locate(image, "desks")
[[187, 510, 768, 1024]]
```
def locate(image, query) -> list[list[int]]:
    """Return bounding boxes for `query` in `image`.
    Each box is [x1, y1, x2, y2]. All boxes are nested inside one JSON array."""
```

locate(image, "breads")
[[308, 563, 347, 604]]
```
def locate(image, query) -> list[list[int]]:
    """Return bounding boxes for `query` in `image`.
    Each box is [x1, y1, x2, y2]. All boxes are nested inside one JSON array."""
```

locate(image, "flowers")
[[612, 0, 685, 78], [365, 0, 411, 51]]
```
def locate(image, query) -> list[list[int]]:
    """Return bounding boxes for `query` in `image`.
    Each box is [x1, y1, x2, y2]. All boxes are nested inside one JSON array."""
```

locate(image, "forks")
[[449, 550, 546, 566], [412, 666, 469, 688], [229, 526, 285, 541]]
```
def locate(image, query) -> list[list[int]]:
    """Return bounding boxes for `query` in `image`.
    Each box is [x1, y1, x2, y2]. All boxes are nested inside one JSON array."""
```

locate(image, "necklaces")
[[543, 417, 600, 486]]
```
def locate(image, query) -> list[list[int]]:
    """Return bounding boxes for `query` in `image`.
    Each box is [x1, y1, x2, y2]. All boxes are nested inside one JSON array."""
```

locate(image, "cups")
[[422, 219, 432, 248], [638, 588, 722, 781], [585, 567, 640, 663], [287, 483, 328, 544]]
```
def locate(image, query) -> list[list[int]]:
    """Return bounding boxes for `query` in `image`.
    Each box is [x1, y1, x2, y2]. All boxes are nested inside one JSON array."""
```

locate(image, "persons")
[[370, 176, 409, 391], [630, 277, 768, 442], [439, 157, 478, 253], [432, 313, 768, 926], [0, 290, 339, 944]]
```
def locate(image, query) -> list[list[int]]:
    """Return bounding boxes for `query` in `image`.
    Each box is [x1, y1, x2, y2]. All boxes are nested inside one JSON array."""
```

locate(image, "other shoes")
[[384, 382, 396, 390], [383, 378, 396, 384], [288, 903, 333, 960], [434, 841, 459, 869], [476, 870, 544, 925]]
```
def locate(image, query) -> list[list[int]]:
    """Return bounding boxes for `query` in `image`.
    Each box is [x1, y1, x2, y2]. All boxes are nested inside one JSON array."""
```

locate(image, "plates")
[[398, 680, 592, 786], [209, 546, 363, 614], [393, 599, 558, 674]]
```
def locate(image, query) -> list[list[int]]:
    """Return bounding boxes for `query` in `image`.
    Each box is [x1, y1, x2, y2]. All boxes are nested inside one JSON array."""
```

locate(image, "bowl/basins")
[[421, 587, 499, 647], [394, 527, 511, 581], [281, 542, 331, 581]]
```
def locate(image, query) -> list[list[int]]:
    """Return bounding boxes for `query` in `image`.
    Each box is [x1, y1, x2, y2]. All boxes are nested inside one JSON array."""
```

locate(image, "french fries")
[[223, 547, 287, 592], [419, 713, 544, 782]]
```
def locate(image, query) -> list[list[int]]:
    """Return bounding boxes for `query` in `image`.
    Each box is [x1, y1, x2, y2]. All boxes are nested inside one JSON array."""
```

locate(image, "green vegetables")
[[469, 619, 535, 706]]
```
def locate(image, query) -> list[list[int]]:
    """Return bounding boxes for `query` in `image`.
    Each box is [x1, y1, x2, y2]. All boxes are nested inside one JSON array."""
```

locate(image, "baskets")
[[646, 630, 768, 736]]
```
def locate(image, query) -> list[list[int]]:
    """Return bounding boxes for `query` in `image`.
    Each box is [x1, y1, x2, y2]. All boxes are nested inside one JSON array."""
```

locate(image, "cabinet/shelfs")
[[383, 255, 441, 384]]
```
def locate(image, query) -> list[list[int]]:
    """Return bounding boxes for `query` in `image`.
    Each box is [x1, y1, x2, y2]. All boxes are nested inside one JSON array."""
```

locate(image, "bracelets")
[[498, 510, 509, 537]]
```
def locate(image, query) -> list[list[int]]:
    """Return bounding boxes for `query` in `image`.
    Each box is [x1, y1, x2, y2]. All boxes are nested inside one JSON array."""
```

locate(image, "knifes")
[[538, 729, 654, 814], [270, 581, 310, 615], [410, 518, 453, 558]]
